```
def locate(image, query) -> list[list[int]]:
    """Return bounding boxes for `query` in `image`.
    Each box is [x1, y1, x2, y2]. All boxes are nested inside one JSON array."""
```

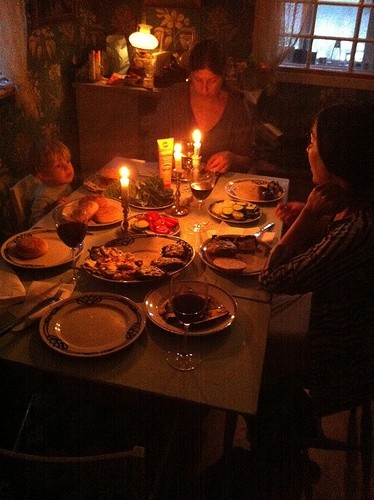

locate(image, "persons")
[[28, 138, 75, 227], [244, 102, 374, 500], [142, 40, 253, 173]]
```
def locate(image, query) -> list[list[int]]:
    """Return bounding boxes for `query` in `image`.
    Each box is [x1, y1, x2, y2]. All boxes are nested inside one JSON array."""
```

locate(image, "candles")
[[192, 129, 202, 158], [173, 143, 182, 171], [118, 166, 130, 208]]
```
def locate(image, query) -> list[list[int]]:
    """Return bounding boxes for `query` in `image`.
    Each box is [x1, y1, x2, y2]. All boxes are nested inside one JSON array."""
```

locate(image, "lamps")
[[128, 23, 159, 51]]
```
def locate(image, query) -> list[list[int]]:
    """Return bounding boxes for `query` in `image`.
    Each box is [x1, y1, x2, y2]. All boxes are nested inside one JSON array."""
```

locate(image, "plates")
[[199, 234, 271, 275], [104, 194, 174, 210], [39, 292, 144, 357], [144, 280, 237, 335], [1, 228, 84, 268], [208, 199, 262, 223], [121, 213, 183, 236], [81, 234, 195, 283], [60, 197, 130, 226]]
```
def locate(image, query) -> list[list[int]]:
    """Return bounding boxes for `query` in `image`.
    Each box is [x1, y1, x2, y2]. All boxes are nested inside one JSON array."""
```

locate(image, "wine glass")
[[56, 206, 88, 292], [166, 271, 208, 371], [188, 164, 214, 233]]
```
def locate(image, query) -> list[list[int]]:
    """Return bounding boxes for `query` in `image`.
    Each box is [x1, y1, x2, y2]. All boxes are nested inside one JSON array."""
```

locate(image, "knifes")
[[0, 298, 59, 337], [254, 223, 273, 237]]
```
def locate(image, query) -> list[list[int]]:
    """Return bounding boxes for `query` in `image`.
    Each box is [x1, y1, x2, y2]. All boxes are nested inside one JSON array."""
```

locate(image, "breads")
[[206, 235, 258, 258], [136, 242, 186, 279], [131, 220, 154, 234], [69, 192, 121, 224], [14, 235, 48, 259], [213, 258, 247, 272]]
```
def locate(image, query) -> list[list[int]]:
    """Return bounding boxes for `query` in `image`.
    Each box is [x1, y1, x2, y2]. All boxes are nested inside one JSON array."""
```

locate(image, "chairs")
[[0, 389, 172, 500], [9, 173, 44, 233]]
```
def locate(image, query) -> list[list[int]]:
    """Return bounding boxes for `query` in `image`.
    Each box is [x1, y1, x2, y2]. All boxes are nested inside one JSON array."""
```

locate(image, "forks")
[[53, 289, 63, 300]]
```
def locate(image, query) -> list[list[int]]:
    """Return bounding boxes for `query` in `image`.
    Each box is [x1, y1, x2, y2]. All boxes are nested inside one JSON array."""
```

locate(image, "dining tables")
[[0, 156, 290, 499]]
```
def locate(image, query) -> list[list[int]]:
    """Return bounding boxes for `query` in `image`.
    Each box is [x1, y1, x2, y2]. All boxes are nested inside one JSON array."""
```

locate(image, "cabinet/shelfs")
[[71, 82, 166, 180]]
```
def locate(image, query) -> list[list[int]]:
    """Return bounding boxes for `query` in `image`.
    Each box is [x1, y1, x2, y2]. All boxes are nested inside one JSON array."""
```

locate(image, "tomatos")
[[137, 211, 178, 234]]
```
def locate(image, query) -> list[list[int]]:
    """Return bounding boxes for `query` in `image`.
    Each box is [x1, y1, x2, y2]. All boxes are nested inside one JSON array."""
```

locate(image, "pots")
[[225, 178, 284, 202]]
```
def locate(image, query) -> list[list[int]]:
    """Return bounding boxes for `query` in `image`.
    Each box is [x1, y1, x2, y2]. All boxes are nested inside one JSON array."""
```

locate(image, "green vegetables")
[[104, 175, 175, 206]]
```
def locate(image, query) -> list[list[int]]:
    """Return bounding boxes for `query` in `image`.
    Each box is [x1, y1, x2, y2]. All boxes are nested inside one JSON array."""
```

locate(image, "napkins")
[[207, 221, 275, 243], [0, 261, 75, 332]]
